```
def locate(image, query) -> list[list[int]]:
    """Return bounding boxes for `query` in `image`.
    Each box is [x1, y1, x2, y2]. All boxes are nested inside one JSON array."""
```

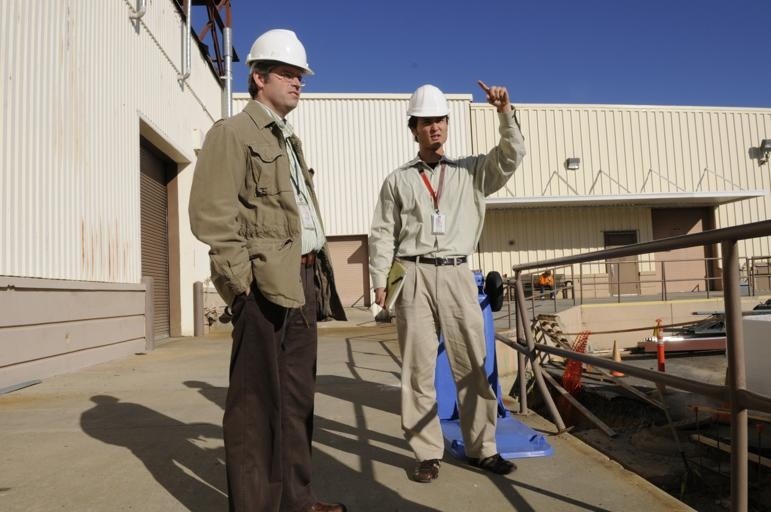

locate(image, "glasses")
[[271, 71, 305, 86]]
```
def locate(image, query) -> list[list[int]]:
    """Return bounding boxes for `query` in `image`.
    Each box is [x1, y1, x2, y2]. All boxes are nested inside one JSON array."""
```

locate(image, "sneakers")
[[413, 459, 441, 484], [469, 453, 516, 475], [302, 500, 347, 512]]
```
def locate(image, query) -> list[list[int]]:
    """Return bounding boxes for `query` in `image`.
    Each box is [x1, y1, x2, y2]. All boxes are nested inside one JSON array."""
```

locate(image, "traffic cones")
[[609, 342, 628, 378]]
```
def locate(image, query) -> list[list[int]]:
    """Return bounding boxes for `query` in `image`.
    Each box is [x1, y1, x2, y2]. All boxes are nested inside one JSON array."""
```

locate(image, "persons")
[[539, 270, 556, 301], [190, 29, 348, 512], [369, 77, 526, 483]]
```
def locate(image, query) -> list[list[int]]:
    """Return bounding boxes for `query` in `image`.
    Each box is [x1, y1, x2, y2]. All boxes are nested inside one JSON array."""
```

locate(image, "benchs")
[[513, 272, 570, 299]]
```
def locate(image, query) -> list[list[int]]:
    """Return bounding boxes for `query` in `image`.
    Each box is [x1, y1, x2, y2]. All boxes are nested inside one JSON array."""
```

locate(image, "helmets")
[[245, 28, 315, 76], [406, 83, 449, 118]]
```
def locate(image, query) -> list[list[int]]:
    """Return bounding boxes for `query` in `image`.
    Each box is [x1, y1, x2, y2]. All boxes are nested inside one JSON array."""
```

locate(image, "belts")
[[301, 253, 319, 266], [401, 255, 469, 267]]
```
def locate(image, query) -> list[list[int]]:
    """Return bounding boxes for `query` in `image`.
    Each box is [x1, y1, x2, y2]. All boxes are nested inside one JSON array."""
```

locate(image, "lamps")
[[760, 137, 771, 167], [565, 156, 582, 171]]
